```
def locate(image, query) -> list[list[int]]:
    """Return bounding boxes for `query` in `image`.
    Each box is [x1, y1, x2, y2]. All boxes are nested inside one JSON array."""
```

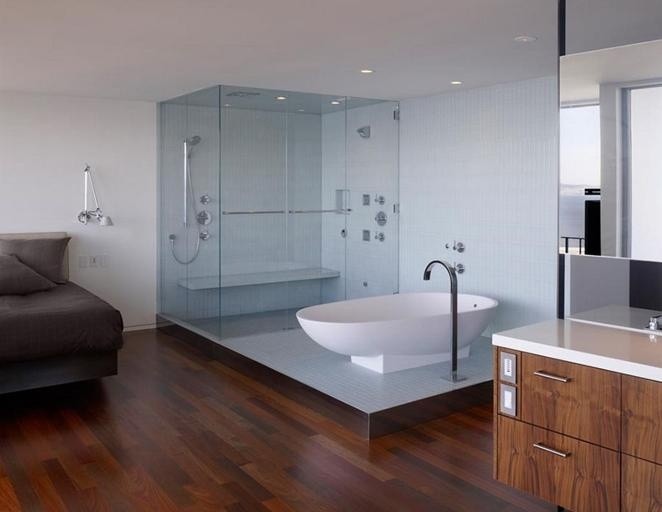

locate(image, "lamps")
[[77, 163, 114, 228]]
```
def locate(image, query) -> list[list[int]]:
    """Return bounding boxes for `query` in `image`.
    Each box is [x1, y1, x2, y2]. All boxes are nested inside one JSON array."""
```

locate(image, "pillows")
[[0, 235, 75, 295]]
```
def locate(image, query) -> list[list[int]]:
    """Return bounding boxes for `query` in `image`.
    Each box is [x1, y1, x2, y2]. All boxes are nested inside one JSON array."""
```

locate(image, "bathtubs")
[[296, 288, 499, 374]]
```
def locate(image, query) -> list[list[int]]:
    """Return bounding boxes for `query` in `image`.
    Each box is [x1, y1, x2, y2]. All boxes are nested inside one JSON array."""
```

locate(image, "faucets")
[[423, 255, 459, 378]]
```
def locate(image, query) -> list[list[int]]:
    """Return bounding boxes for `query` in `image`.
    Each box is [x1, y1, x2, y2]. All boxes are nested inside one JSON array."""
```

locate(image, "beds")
[[1, 230, 124, 397]]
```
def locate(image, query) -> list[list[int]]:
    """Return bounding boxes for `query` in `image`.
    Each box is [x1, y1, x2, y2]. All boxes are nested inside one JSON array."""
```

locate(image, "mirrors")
[[552, 1, 661, 336]]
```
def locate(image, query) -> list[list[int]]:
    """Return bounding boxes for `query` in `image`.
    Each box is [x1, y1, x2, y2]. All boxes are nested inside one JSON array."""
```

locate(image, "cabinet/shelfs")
[[493, 348, 662, 512]]
